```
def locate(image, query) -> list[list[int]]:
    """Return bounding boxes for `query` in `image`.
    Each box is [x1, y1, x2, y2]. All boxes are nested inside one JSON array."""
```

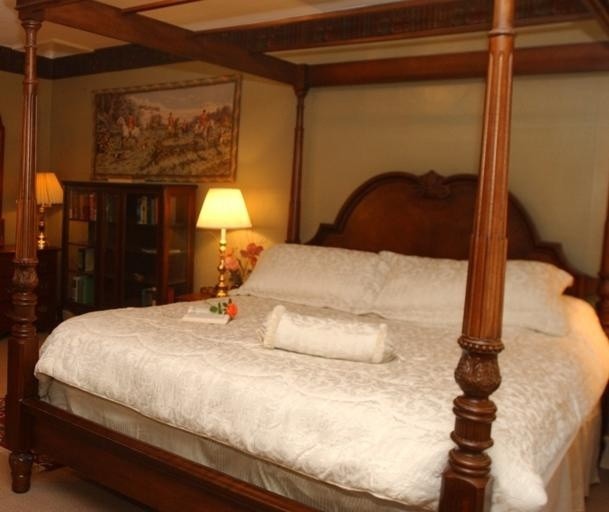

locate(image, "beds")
[[8, 2, 609, 510]]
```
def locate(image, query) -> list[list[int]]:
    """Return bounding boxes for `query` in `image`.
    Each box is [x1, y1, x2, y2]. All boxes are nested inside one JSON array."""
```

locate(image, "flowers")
[[223, 244, 263, 289], [204, 299, 238, 324]]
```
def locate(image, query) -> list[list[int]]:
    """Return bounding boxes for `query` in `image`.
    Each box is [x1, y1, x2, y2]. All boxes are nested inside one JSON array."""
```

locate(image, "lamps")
[[16, 171, 64, 251], [195, 186, 252, 298]]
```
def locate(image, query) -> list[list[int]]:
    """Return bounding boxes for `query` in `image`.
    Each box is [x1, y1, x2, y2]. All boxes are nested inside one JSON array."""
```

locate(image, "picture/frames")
[[91, 72, 244, 182]]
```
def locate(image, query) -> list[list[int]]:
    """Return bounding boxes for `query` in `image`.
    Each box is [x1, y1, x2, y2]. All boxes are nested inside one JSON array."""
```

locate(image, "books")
[[182, 305, 231, 325], [68, 272, 94, 305], [133, 194, 177, 226], [66, 189, 97, 221], [76, 246, 95, 272]]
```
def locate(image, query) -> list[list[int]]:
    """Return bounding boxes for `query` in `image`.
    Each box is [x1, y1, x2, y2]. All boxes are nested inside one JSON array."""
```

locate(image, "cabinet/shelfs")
[[57, 178, 199, 316], [0, 245, 62, 338]]
